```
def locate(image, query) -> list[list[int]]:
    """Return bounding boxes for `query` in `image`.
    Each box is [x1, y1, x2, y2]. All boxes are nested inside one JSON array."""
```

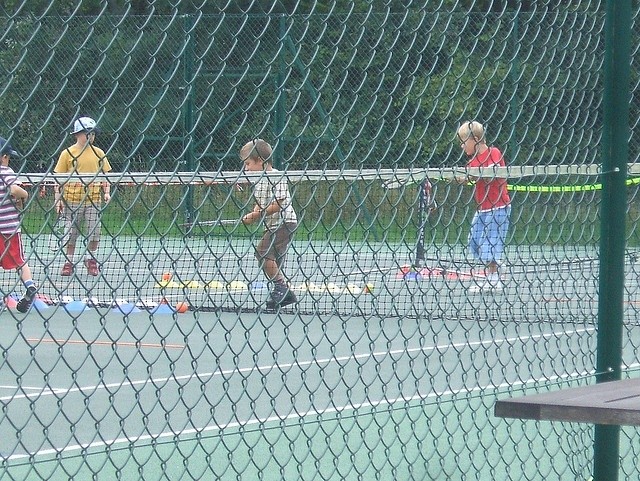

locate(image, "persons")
[[0, 138, 38, 312], [54, 117, 113, 277], [240, 140, 298, 309], [453, 121, 511, 292]]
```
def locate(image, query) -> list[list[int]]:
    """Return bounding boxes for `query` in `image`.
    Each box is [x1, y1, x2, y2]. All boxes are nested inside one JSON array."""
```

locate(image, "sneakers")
[[469, 278, 490, 294], [16, 286, 38, 313], [265, 283, 292, 308], [278, 289, 297, 307], [0, 296, 20, 312], [60, 263, 75, 275], [482, 280, 506, 294], [83, 255, 99, 276]]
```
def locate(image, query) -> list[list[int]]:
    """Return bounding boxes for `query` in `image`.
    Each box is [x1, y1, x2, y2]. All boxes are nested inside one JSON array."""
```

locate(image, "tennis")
[[364, 283, 375, 294], [176, 303, 187, 313], [162, 273, 173, 282], [161, 297, 169, 304]]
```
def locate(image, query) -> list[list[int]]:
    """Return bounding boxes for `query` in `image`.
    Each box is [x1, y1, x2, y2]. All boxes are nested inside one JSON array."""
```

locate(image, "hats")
[[0, 136, 23, 157], [69, 116, 98, 134]]
